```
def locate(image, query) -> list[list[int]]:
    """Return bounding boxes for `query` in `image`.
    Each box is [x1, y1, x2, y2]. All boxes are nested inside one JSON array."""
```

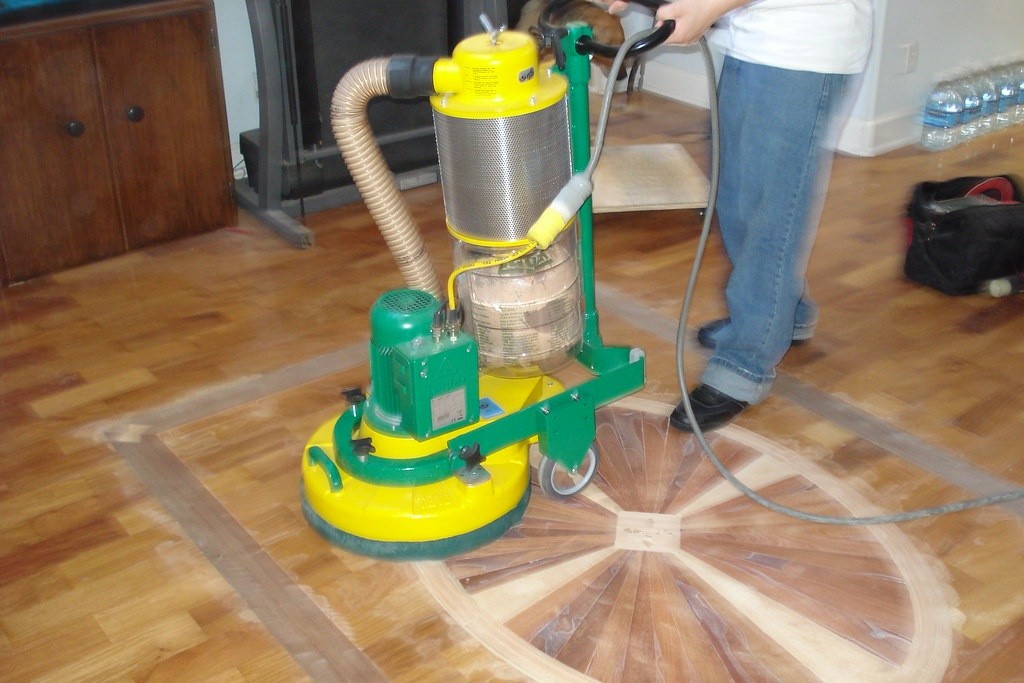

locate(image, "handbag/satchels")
[[903, 174, 1024, 296]]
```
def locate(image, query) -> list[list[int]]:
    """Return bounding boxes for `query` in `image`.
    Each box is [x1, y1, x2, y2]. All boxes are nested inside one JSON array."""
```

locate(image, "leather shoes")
[[670, 380, 749, 431], [698, 315, 797, 348]]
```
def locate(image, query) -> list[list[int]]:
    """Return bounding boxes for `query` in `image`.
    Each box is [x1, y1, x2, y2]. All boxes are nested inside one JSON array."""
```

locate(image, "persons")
[[600, 0, 876, 433]]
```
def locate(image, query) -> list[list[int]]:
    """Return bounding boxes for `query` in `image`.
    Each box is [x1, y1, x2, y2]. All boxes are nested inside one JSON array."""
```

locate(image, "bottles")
[[920, 54, 1024, 152]]
